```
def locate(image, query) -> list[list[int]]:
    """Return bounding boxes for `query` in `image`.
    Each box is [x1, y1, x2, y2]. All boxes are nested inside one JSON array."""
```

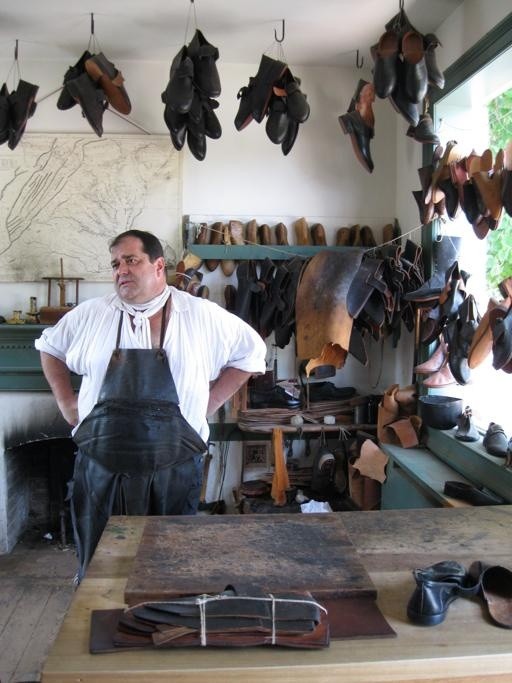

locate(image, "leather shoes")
[[407, 559, 481, 625], [160, 30, 223, 161], [56, 36, 131, 139], [350, 79, 375, 140], [176, 220, 512, 407], [412, 139, 512, 238], [233, 54, 310, 157], [369, 12, 445, 143], [455, 405, 481, 440], [1, 80, 40, 149], [484, 421, 508, 456], [338, 111, 375, 173], [446, 479, 505, 505]]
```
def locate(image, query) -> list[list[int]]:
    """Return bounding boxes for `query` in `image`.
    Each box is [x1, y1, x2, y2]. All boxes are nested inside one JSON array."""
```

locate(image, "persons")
[[31, 227, 268, 598]]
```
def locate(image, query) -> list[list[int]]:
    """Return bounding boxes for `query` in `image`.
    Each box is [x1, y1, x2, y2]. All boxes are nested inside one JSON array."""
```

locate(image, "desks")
[[38, 503, 511, 682]]
